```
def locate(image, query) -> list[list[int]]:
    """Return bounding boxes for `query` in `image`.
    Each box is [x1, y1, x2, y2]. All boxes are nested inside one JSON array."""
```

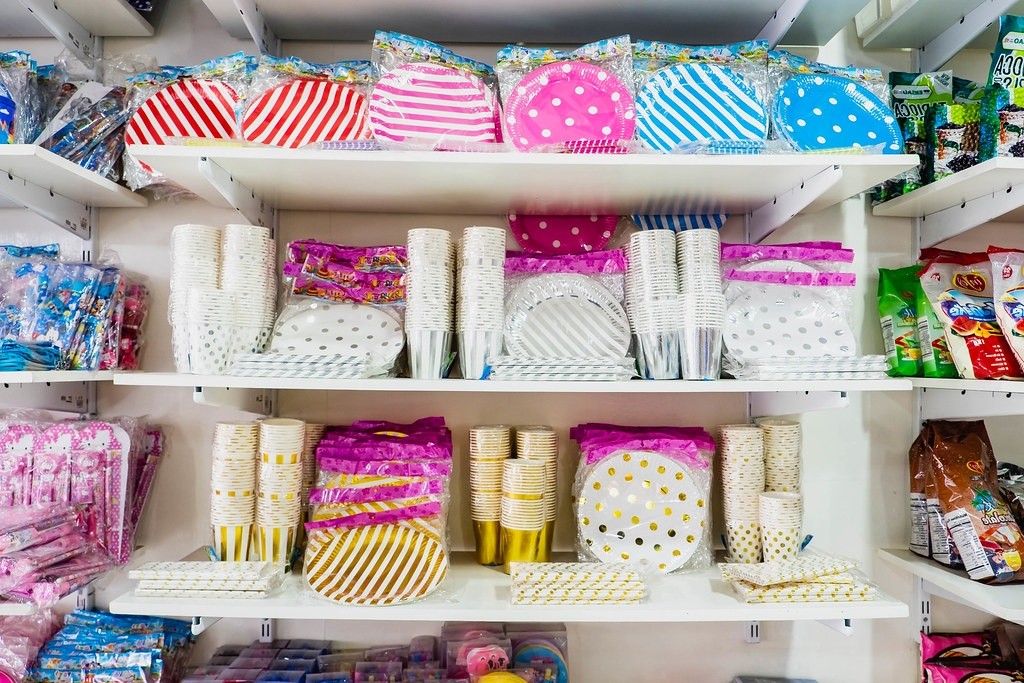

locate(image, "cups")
[[716, 415, 803, 564], [210, 418, 325, 562], [168, 222, 278, 376], [404, 227, 506, 379], [469, 425, 558, 575], [621, 229, 727, 381]]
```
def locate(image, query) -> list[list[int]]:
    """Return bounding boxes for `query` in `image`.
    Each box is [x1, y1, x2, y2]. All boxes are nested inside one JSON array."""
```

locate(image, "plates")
[[723, 259, 857, 365], [305, 432, 449, 607], [504, 272, 631, 357], [578, 450, 704, 574], [271, 305, 403, 367], [122, 60, 905, 174]]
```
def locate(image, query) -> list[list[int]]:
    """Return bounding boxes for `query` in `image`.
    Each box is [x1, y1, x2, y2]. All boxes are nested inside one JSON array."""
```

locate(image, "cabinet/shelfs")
[[0, 0, 1024, 683]]
